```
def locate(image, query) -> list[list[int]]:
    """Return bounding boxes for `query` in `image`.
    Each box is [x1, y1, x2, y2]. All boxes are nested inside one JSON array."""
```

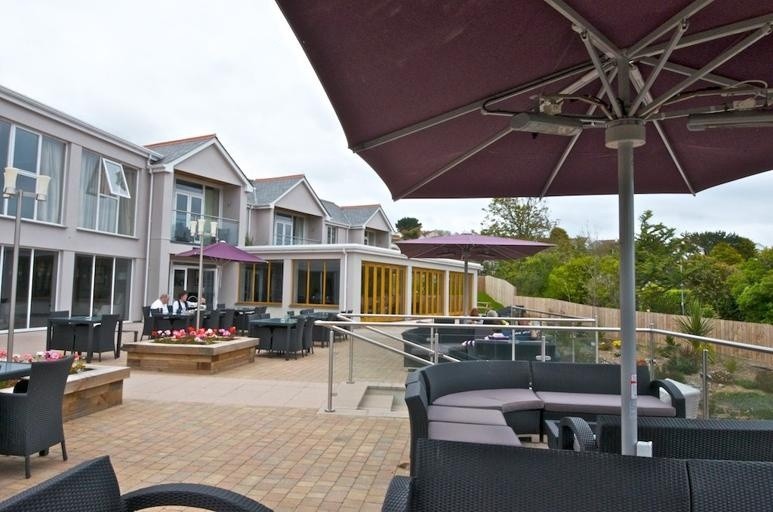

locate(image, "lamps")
[[686, 92, 773, 131], [510, 100, 584, 137]]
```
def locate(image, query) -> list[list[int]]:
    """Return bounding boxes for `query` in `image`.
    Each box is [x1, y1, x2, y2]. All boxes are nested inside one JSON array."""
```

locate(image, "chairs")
[[1, 454, 276, 512], [0, 355, 74, 478]]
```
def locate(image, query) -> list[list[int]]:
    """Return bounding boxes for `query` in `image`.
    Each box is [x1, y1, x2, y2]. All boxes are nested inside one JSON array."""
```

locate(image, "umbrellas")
[[176, 240, 269, 311], [277, 1, 772, 456], [394, 233, 559, 316]]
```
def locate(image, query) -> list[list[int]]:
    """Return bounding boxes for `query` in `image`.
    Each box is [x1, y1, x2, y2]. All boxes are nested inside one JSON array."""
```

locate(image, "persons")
[[149, 292, 170, 318], [466, 307, 483, 324], [483, 310, 501, 324], [172, 291, 188, 316]]
